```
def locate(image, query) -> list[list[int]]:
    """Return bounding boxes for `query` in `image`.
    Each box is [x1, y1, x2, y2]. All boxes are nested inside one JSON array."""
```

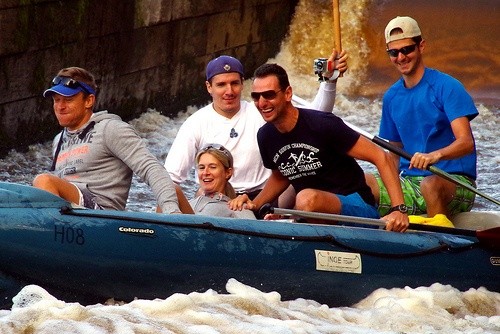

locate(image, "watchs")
[[390, 204, 408, 212]]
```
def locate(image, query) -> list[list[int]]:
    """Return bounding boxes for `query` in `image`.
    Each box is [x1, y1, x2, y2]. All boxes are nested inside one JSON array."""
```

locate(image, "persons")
[[31, 66, 182, 214], [362, 15, 479, 217], [155, 48, 349, 214], [156, 143, 257, 220], [227, 63, 410, 233]]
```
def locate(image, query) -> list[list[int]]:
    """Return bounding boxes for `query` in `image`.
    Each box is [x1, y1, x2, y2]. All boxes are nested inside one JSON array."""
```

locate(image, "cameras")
[[314, 58, 328, 72]]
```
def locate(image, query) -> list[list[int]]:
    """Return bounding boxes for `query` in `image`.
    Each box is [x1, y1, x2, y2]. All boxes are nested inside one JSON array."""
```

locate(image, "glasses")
[[50, 76, 79, 89], [251, 90, 281, 101], [386, 43, 415, 57], [200, 144, 228, 156]]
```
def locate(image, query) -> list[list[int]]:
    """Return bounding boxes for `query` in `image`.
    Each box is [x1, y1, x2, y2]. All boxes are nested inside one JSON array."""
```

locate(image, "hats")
[[42, 78, 95, 98], [206, 55, 244, 81], [384, 16, 422, 44]]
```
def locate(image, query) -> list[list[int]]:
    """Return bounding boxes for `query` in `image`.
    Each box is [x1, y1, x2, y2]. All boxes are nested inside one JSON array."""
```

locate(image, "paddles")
[[259, 204, 500, 258], [292, 93, 500, 206]]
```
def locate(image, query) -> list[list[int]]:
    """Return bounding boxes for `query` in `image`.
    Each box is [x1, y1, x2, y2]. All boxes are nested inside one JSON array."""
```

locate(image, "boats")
[[0, 182, 500, 308]]
